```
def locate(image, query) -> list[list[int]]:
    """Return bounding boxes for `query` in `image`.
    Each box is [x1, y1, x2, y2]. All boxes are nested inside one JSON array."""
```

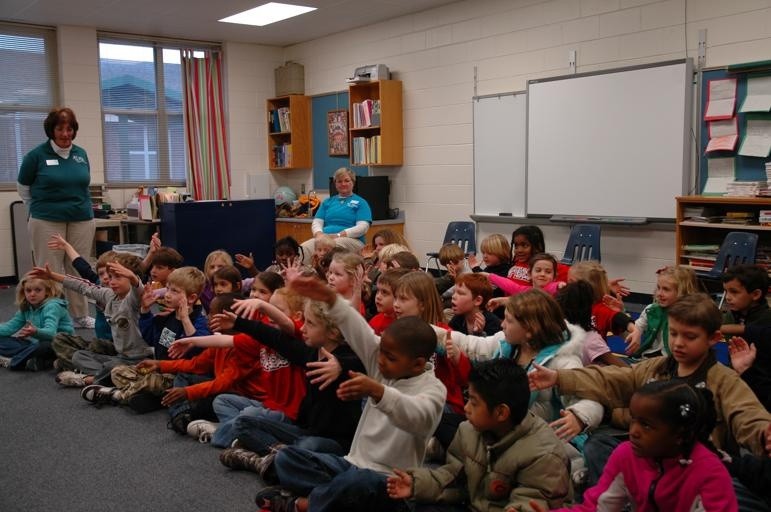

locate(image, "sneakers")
[[54, 359, 64, 370], [221, 448, 257, 471], [255, 485, 298, 509], [187, 420, 220, 443], [130, 392, 169, 412], [80, 386, 129, 406], [56, 371, 95, 388]]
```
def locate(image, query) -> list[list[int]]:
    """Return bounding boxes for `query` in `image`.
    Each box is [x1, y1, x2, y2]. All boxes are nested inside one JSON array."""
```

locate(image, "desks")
[[275, 216, 405, 245], [94, 216, 126, 245], [111, 214, 161, 244]]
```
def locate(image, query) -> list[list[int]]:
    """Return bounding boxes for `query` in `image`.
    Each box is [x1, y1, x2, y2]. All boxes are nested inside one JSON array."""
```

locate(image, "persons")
[[0, 225, 771, 510], [17, 107, 97, 330], [297, 167, 373, 266]]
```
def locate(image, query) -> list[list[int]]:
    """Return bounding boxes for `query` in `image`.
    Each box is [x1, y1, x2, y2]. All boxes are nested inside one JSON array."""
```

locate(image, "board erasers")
[[499, 213, 512, 216]]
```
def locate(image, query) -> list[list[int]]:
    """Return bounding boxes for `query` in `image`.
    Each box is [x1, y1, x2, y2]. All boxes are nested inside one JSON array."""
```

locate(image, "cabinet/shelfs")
[[349, 80, 403, 166], [266, 95, 311, 170], [676, 196, 770, 270]]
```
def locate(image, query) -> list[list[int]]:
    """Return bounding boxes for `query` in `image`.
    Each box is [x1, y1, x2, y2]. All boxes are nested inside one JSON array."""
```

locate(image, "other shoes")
[[173, 413, 218, 435], [2, 358, 11, 368], [27, 358, 49, 371]]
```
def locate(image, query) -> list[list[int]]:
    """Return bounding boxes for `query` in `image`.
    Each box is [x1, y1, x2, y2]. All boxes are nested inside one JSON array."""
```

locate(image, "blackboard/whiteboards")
[[524, 57, 693, 225], [469, 70, 700, 231]]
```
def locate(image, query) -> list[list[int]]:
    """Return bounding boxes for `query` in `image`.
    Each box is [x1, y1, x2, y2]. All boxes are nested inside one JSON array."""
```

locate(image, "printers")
[[345, 64, 392, 83]]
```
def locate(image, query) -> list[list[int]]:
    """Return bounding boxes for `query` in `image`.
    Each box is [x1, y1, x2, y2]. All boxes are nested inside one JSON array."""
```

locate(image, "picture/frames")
[[327, 110, 349, 157]]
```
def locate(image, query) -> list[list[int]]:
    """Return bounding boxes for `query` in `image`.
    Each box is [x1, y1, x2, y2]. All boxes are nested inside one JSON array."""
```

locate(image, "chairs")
[[559, 221, 602, 266], [425, 220, 476, 275], [695, 231, 759, 310]]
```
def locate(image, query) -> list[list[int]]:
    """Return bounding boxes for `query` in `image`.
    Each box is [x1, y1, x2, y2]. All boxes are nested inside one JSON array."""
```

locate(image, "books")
[[678, 161, 771, 310], [269, 106, 292, 168], [352, 98, 382, 164], [137, 183, 182, 223]]
